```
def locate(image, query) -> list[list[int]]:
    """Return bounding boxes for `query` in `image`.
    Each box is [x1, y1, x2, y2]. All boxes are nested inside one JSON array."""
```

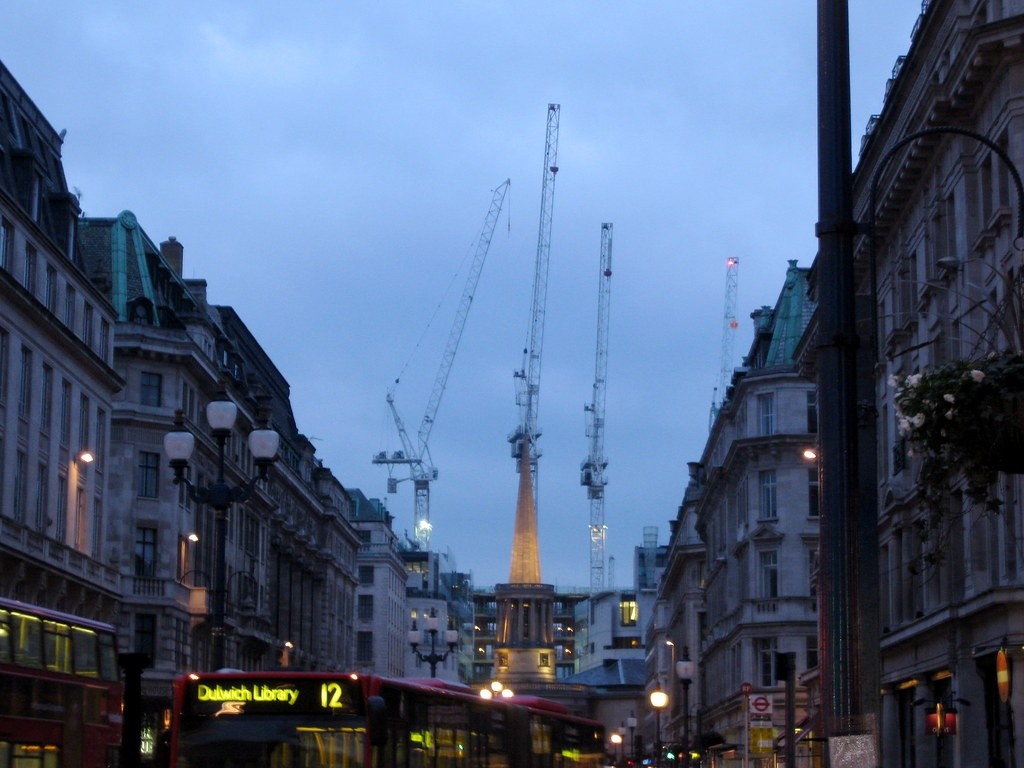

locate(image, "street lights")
[[618, 721, 627, 761], [650, 687, 669, 768], [675, 646, 695, 768], [611, 735, 622, 758], [628, 709, 637, 758]]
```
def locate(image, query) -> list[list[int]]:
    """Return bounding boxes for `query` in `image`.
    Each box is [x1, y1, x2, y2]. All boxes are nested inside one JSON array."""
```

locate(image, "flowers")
[[888, 348, 1024, 574]]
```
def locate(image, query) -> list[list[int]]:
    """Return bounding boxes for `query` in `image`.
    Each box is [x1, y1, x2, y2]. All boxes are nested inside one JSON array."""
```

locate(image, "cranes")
[[503, 102, 563, 583], [707, 256, 740, 439], [580, 221, 614, 595], [372, 177, 512, 553]]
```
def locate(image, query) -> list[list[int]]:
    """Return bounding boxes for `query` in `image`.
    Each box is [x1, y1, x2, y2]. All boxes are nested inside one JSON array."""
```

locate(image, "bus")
[[169, 671, 607, 768], [0, 595, 128, 768]]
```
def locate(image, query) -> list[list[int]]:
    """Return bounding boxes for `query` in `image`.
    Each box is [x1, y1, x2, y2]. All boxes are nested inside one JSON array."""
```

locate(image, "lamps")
[[803, 444, 821, 458], [283, 642, 294, 650], [184, 533, 198, 543], [75, 451, 93, 467]]
